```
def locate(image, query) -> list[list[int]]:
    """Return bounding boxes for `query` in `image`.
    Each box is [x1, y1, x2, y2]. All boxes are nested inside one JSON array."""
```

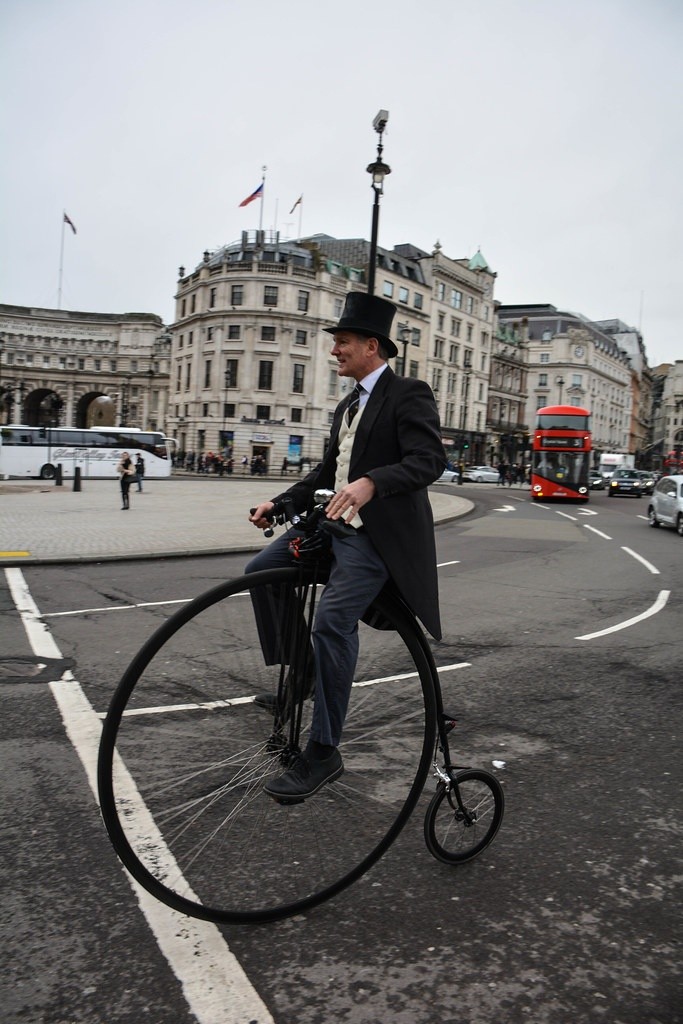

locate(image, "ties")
[[348, 383, 364, 428]]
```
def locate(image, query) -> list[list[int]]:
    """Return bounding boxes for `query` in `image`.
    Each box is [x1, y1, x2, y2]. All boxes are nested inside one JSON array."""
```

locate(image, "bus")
[[663, 451, 683, 476], [0, 423, 181, 480], [530, 405, 593, 502]]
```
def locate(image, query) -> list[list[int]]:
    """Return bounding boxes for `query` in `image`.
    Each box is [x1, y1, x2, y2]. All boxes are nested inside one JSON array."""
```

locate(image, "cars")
[[608, 469, 663, 498], [436, 468, 460, 482], [647, 475, 683, 535], [588, 470, 604, 490]]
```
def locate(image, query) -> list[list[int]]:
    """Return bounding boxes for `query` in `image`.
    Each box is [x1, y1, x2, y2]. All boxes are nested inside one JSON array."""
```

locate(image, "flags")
[[289, 197, 301, 214], [64, 214, 77, 235], [240, 184, 263, 207]]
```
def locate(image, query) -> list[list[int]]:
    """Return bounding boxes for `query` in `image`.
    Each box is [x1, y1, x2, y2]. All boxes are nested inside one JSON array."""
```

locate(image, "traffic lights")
[[464, 441, 469, 449]]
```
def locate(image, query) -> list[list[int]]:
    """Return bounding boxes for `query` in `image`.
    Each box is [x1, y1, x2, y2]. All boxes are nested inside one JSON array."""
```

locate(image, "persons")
[[280, 457, 289, 475], [134, 453, 145, 493], [197, 451, 234, 477], [240, 454, 267, 476], [248, 291, 448, 801], [497, 460, 528, 485], [117, 451, 136, 510], [537, 458, 554, 470], [170, 447, 196, 472]]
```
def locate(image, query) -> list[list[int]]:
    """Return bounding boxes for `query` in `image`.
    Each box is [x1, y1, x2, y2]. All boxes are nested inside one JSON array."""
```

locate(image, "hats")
[[322, 292, 398, 358]]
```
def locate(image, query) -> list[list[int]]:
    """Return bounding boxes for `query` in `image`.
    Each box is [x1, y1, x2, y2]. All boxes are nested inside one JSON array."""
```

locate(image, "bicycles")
[[97, 489, 504, 927]]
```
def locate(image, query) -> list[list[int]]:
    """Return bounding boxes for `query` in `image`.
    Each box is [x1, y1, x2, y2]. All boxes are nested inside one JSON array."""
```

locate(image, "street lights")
[[401, 321, 413, 376], [220, 369, 232, 476], [367, 157, 391, 295], [458, 358, 473, 485], [558, 378, 566, 405]]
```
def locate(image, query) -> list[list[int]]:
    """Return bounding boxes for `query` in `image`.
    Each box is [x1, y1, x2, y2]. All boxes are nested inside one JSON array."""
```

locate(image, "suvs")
[[462, 466, 500, 482]]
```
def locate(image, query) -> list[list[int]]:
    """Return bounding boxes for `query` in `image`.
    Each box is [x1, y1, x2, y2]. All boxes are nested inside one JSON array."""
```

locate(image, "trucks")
[[597, 453, 635, 486]]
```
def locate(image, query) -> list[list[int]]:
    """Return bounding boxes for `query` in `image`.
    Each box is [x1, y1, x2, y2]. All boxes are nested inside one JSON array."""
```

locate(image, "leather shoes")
[[253, 672, 318, 710], [262, 747, 344, 801]]
